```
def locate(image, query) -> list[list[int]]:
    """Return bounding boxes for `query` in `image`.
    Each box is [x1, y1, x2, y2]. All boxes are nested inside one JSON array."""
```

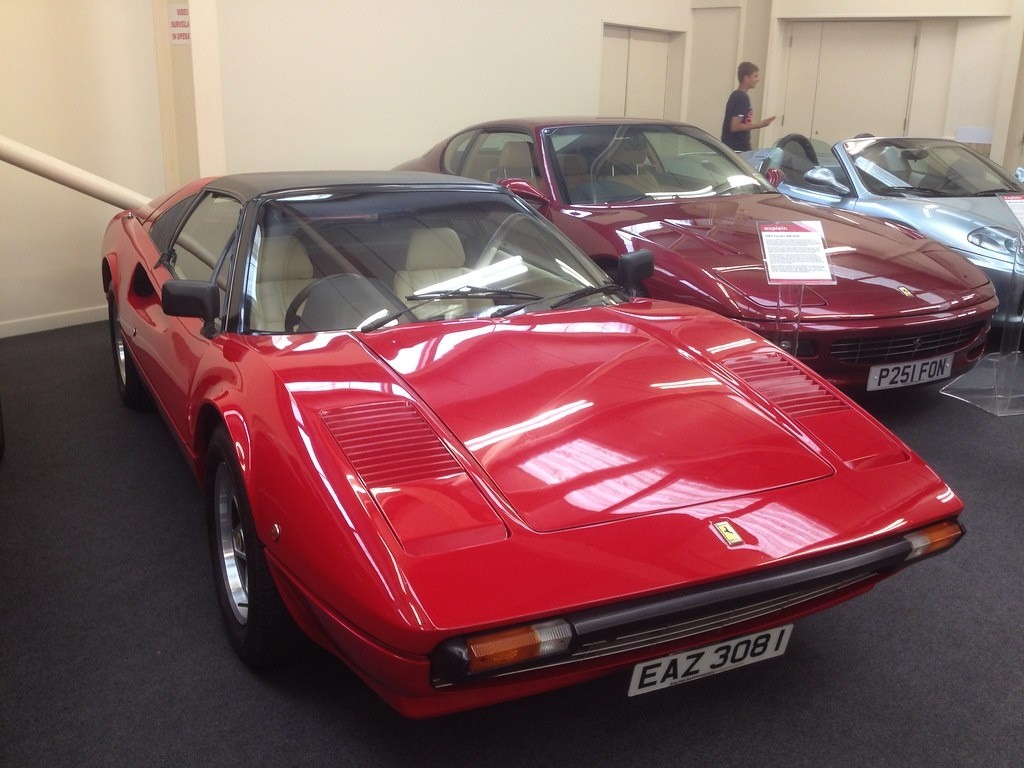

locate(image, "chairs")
[[558, 154, 598, 204], [389, 227, 496, 323], [249, 235, 315, 332], [596, 135, 660, 205], [497, 140, 541, 190]]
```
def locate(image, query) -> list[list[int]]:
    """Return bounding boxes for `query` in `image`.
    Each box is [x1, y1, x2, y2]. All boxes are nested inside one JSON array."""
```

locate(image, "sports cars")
[[100, 169, 967, 723], [661, 131, 1024, 352], [359, 111, 1004, 406]]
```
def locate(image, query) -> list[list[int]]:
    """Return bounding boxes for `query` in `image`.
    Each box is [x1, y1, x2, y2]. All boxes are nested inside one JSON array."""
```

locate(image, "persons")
[[721, 62, 775, 150]]
